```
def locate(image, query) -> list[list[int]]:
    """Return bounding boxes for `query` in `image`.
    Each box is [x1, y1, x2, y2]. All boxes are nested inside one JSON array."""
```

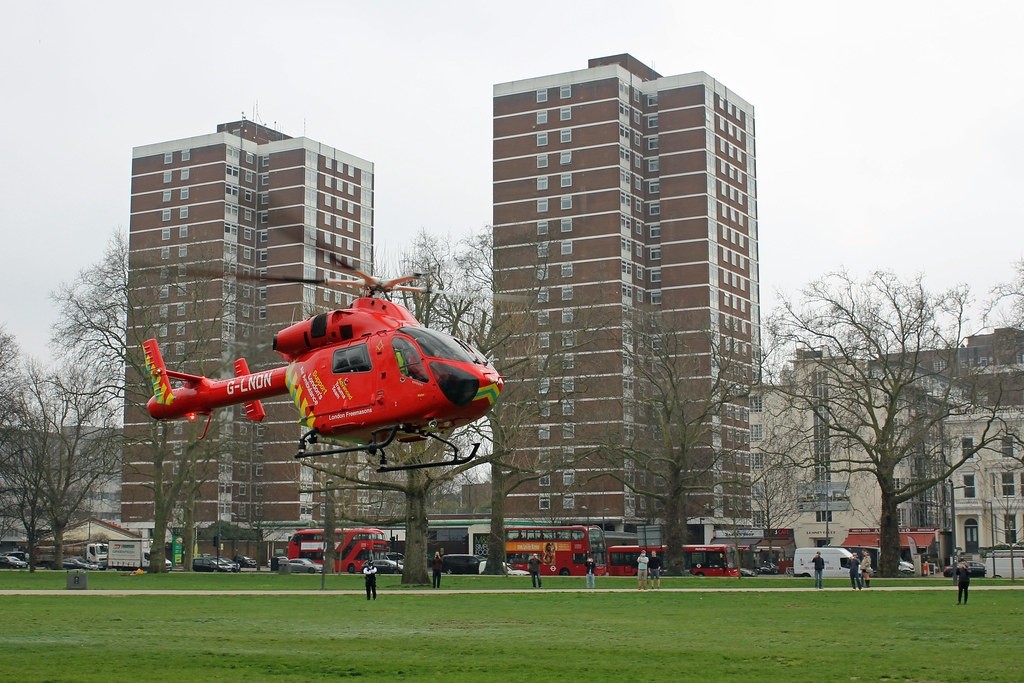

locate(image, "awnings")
[[712, 538, 792, 550], [841, 533, 934, 548]]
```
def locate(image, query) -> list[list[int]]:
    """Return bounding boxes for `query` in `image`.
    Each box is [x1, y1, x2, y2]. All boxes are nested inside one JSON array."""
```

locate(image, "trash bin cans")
[[923, 563, 928, 576], [271, 557, 279, 572]]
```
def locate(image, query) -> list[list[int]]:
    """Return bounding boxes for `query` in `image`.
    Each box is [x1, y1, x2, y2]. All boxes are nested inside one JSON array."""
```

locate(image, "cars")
[[0, 555, 28, 570], [380, 552, 405, 569], [502, 562, 530, 576], [55, 556, 99, 571], [207, 556, 241, 572], [192, 558, 233, 572], [943, 561, 987, 578], [267, 556, 289, 569], [740, 568, 758, 577], [361, 559, 403, 574], [232, 555, 257, 568], [289, 558, 323, 574]]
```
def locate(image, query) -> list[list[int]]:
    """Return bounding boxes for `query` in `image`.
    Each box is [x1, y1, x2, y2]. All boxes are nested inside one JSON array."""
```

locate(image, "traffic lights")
[[213, 535, 218, 547]]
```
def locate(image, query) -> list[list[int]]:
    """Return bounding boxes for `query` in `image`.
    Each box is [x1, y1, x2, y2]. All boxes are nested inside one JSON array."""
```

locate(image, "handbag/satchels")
[[866, 567, 872, 572]]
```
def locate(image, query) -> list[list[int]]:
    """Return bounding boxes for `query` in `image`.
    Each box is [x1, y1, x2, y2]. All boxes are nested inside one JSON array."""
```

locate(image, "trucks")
[[108, 538, 173, 573], [86, 543, 109, 571], [822, 545, 916, 575]]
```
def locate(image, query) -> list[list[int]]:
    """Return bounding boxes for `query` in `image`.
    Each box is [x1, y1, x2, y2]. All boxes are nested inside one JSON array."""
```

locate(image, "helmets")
[[367, 560, 374, 566]]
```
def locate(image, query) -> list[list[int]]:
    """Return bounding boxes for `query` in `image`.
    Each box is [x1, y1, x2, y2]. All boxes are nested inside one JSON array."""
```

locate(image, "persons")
[[407, 336, 451, 383], [860, 550, 872, 588], [648, 550, 661, 589], [432, 551, 444, 588], [637, 550, 649, 590], [363, 560, 377, 600], [584, 557, 595, 589], [528, 553, 543, 588], [848, 552, 862, 591], [956, 562, 971, 605], [812, 551, 825, 588]]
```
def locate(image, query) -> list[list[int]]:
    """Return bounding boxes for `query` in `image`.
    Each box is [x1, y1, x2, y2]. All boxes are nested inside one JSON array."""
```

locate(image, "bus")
[[288, 527, 395, 574], [608, 543, 740, 577], [504, 524, 608, 576]]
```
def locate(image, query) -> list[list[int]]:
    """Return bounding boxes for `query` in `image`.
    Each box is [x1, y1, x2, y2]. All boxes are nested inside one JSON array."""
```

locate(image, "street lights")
[[984, 500, 996, 578]]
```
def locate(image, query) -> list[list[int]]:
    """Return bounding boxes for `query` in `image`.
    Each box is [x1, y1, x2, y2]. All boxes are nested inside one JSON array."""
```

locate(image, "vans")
[[441, 554, 488, 575], [1, 552, 25, 561], [794, 547, 866, 578]]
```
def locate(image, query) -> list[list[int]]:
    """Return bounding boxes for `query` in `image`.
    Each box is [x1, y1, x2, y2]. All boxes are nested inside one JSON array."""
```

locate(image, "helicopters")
[[130, 194, 509, 474]]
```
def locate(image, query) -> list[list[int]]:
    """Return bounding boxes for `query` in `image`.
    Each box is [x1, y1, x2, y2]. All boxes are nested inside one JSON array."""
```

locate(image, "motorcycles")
[[753, 560, 780, 575]]
